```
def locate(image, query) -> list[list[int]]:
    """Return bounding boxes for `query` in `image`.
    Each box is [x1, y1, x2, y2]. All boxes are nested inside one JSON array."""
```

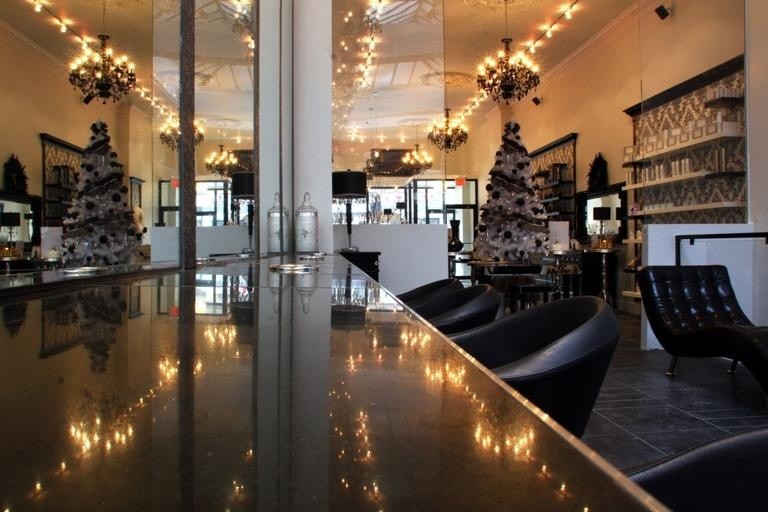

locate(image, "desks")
[[583, 247, 621, 308], [338, 250, 382, 283], [0, 252, 672, 512], [553, 249, 581, 295], [466, 260, 510, 276], [0, 253, 280, 291]]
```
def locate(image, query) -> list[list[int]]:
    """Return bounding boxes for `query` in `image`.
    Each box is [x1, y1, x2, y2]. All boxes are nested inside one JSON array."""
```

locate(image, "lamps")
[[230, 170, 254, 254], [0, 211, 21, 256], [424, 348, 468, 393], [203, 323, 237, 351], [158, 341, 203, 380], [401, 326, 431, 356], [400, 143, 433, 177], [68, 34, 136, 105], [395, 201, 407, 223], [593, 206, 610, 235], [67, 389, 136, 459], [332, 168, 367, 253], [205, 145, 238, 177], [615, 207, 621, 235], [476, 37, 540, 105], [472, 396, 537, 471], [229, 263, 254, 327], [426, 107, 468, 154], [383, 208, 392, 224], [159, 108, 205, 152], [331, 263, 366, 333]]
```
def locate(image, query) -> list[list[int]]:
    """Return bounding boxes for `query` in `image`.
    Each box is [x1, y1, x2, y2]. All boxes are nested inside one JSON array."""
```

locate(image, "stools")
[[509, 281, 551, 314]]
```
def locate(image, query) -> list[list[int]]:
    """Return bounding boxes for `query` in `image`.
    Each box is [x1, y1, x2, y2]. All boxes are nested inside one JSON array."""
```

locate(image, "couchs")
[[395, 277, 464, 306], [627, 424, 768, 512], [636, 264, 768, 394], [411, 282, 500, 335], [445, 295, 620, 439]]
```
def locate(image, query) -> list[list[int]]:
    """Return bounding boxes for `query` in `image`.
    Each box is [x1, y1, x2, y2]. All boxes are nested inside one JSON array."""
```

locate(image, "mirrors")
[[0, 199, 35, 246], [581, 190, 622, 236]]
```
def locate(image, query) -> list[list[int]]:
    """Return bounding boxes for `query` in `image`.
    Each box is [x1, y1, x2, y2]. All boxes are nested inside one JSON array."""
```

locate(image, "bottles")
[[294, 191, 318, 254], [266, 191, 289, 254]]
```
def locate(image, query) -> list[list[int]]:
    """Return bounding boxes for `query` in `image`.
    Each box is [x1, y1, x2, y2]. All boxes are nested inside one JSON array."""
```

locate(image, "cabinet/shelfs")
[[37, 292, 84, 360], [38, 131, 85, 227], [621, 53, 748, 300], [528, 133, 576, 232]]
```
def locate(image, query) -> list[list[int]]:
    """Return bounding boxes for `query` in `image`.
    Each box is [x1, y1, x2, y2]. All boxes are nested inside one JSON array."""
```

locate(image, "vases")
[[448, 219, 463, 252]]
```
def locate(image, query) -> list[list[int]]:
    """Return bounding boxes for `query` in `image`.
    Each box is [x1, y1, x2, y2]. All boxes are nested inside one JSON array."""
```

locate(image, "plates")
[[63, 266, 108, 273], [268, 262, 319, 274]]
